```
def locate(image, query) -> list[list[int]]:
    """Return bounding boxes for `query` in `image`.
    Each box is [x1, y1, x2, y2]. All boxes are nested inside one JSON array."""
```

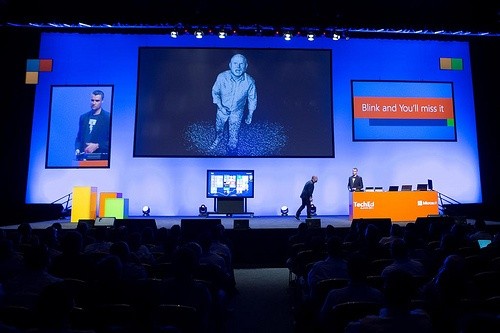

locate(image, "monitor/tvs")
[[206, 170, 254, 198], [402, 185, 412, 191], [428, 179, 433, 190], [389, 186, 399, 191], [417, 184, 427, 190]]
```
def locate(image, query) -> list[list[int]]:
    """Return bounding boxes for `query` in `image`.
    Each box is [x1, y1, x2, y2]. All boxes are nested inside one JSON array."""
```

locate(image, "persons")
[[288, 217, 500, 333], [209, 53, 257, 155], [348, 168, 363, 191], [75, 90, 110, 160], [295, 176, 318, 220], [0, 222, 233, 333]]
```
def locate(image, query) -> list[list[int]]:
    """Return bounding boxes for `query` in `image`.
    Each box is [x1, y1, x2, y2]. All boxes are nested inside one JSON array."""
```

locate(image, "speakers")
[[304, 219, 321, 228], [234, 220, 250, 229], [180, 218, 221, 230], [415, 216, 456, 227], [351, 218, 392, 231], [76, 217, 157, 231]]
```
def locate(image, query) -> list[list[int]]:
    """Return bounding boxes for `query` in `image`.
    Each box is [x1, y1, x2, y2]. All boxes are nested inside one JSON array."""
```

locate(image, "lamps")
[[311, 204, 318, 216], [199, 204, 207, 217], [142, 206, 151, 216], [281, 206, 288, 216], [169, 24, 350, 40]]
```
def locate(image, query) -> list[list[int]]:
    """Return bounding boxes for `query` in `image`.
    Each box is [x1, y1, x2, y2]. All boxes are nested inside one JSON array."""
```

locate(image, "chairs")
[[286, 241, 500, 333], [0, 238, 226, 333]]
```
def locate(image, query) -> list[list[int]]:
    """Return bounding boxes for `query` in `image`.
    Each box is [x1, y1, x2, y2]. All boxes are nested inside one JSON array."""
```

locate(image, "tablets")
[[478, 240, 492, 249]]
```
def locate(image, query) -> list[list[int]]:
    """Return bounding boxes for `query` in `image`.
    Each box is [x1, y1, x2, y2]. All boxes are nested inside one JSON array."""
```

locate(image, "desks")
[[348, 191, 440, 222]]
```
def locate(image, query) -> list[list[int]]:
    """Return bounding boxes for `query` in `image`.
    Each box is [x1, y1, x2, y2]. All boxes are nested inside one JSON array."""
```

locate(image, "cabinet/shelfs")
[[206, 198, 254, 217]]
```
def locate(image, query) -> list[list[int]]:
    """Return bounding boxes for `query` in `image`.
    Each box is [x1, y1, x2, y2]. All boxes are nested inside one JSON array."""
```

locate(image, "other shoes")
[[296, 217, 300, 220]]
